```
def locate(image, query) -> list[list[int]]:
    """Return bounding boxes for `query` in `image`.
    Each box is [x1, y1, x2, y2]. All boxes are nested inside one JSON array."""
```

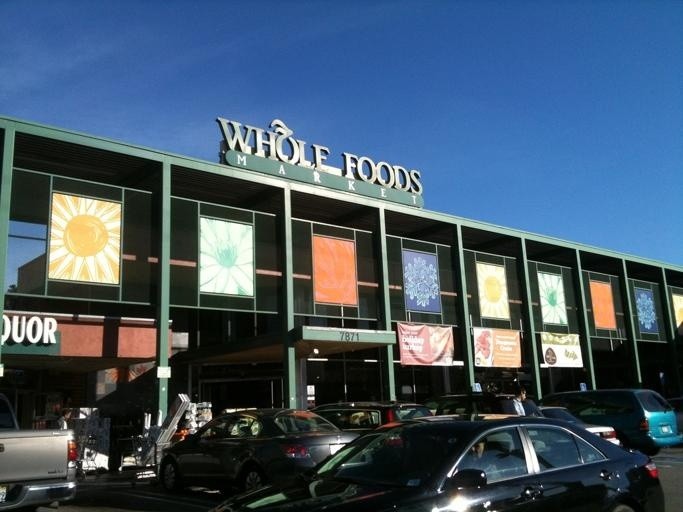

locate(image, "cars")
[[538, 406, 620, 460], [159, 408, 374, 500], [309, 401, 435, 465], [209, 414, 666, 512]]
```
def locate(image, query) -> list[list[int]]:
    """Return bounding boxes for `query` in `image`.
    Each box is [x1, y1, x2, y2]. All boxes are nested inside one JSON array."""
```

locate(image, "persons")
[[56, 409, 72, 430], [459, 438, 500, 482], [506, 388, 527, 416]]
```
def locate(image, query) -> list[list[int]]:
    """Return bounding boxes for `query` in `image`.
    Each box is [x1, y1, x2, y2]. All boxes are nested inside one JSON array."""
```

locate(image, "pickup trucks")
[[1, 394, 80, 512]]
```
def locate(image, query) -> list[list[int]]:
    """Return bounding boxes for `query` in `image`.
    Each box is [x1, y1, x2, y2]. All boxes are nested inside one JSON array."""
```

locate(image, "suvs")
[[434, 393, 547, 451], [537, 387, 683, 455]]
